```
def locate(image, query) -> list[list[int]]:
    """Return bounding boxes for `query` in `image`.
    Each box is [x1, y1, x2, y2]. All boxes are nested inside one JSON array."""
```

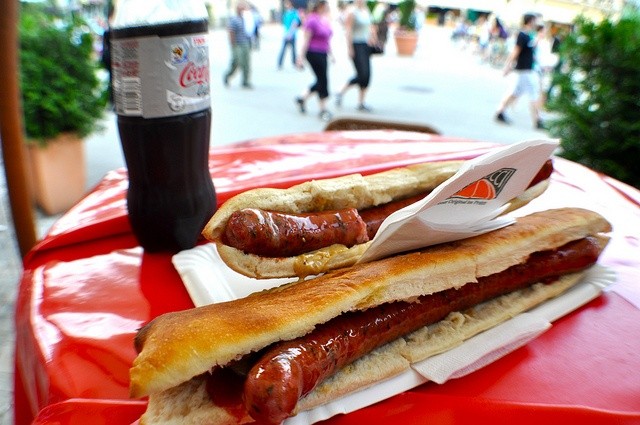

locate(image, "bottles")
[[105, 0, 220, 256]]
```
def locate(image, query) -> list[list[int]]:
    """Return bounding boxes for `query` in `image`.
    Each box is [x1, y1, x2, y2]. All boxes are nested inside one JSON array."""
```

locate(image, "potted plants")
[[396, 2, 418, 57], [20, 12, 110, 218]]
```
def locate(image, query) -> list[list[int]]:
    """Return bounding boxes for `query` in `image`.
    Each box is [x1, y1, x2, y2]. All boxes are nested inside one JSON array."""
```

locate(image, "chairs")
[[323, 117, 438, 138]]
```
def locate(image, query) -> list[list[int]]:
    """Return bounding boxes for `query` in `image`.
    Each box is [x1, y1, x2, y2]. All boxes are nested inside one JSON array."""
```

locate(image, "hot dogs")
[[202, 148, 550, 280], [131, 207, 613, 425]]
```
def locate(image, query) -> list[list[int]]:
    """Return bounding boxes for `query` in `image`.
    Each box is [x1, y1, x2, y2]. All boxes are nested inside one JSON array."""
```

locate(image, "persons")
[[551, 23, 559, 55], [294, 0, 337, 124], [435, 7, 490, 75], [336, 0, 352, 38], [530, 10, 547, 94], [277, 0, 301, 68], [494, 9, 551, 132], [480, 16, 510, 70], [373, 4, 401, 54], [336, 0, 378, 114], [222, 0, 257, 91]]
